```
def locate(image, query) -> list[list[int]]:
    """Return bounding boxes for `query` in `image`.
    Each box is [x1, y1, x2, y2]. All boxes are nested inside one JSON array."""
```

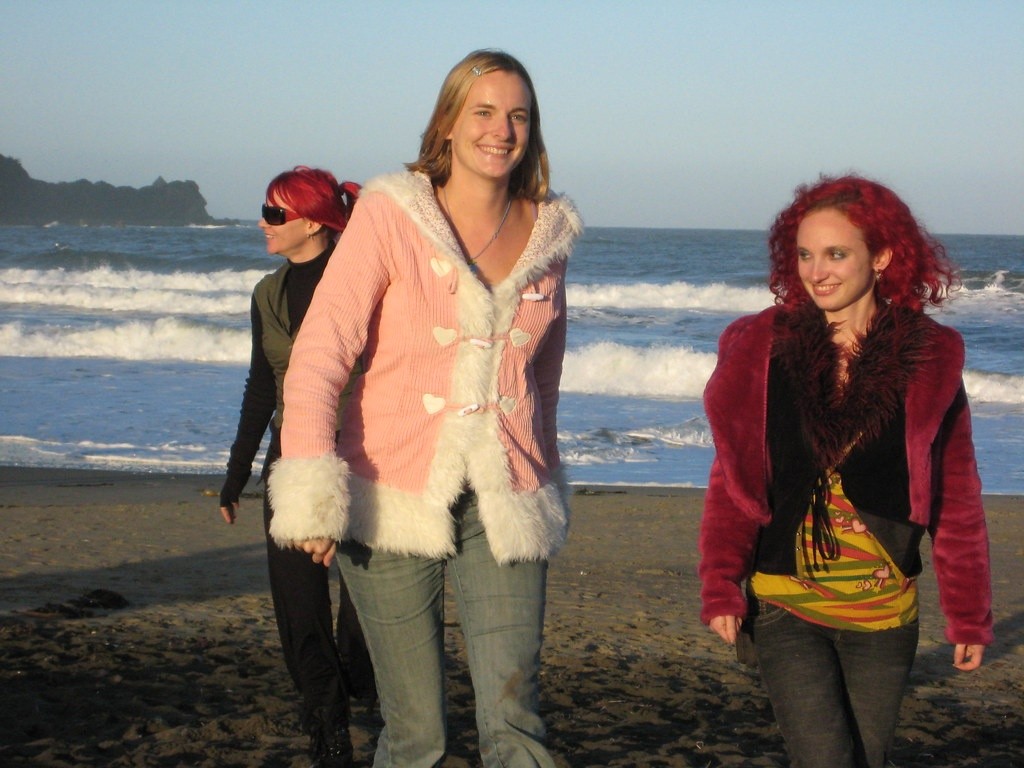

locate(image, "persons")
[[697, 175, 996, 768], [218, 164, 386, 768], [268, 49, 577, 768]]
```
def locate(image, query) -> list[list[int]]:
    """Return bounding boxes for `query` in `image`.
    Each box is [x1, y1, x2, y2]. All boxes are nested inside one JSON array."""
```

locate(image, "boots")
[[306, 713, 353, 768]]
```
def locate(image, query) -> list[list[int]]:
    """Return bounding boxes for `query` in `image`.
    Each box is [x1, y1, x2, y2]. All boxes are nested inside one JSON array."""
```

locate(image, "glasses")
[[261, 204, 302, 226]]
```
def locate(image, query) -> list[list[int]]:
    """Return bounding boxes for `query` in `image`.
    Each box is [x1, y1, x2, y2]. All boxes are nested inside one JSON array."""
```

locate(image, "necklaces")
[[439, 184, 513, 264]]
